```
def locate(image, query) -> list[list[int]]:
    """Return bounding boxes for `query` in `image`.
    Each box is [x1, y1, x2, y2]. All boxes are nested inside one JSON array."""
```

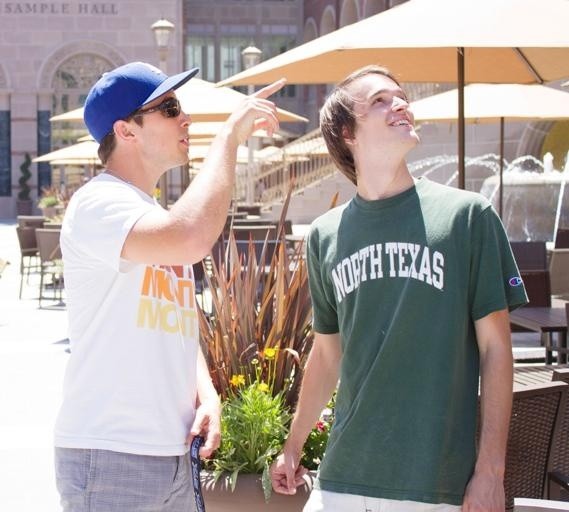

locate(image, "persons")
[[53, 61, 288, 512], [269, 66, 530, 512]]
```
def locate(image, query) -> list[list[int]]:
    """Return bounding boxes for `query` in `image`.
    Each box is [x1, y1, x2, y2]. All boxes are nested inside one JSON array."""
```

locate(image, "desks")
[[510, 307, 566, 366]]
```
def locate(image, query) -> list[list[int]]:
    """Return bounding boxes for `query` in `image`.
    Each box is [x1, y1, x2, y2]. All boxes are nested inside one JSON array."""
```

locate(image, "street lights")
[[147, 12, 176, 209], [240, 38, 264, 210]]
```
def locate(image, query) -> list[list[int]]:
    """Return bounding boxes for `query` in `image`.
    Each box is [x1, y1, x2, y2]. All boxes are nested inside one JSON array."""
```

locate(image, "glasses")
[[108, 97, 181, 136]]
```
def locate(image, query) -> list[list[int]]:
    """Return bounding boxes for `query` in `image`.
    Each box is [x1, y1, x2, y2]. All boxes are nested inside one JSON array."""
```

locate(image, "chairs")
[[510, 268, 559, 364], [473, 385, 564, 512], [513, 240, 548, 268], [546, 369, 569, 501], [13, 202, 305, 309]]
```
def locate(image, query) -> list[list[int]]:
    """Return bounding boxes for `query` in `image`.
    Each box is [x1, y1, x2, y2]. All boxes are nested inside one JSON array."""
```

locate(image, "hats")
[[83, 62, 200, 144]]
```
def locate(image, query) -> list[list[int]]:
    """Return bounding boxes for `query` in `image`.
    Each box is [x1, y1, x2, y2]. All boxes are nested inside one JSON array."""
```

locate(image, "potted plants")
[[197, 353, 330, 511]]
